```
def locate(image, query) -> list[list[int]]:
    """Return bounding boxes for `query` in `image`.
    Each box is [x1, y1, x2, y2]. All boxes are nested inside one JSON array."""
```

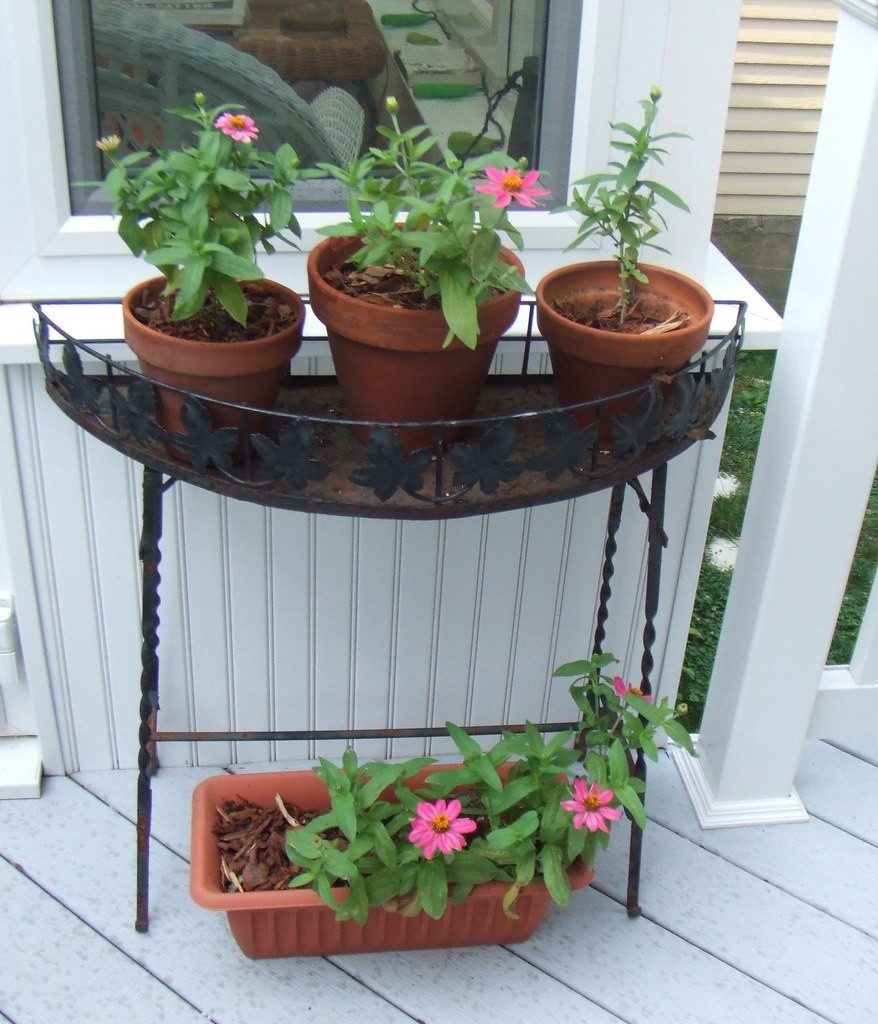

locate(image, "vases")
[[121, 275, 306, 465], [535, 260, 715, 440], [188, 761, 593, 960], [306, 223, 526, 458]]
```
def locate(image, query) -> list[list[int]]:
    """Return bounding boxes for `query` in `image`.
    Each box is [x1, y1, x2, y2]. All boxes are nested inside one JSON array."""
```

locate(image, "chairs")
[[90, 0, 366, 182]]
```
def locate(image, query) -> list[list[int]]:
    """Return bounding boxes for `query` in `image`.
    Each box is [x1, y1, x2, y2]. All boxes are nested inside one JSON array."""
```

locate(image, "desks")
[[102, 0, 386, 158]]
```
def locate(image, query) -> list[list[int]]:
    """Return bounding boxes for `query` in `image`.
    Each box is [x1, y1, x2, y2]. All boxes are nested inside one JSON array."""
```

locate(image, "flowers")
[[315, 97, 554, 352], [70, 91, 306, 326], [285, 654, 698, 919], [549, 88, 695, 317]]
